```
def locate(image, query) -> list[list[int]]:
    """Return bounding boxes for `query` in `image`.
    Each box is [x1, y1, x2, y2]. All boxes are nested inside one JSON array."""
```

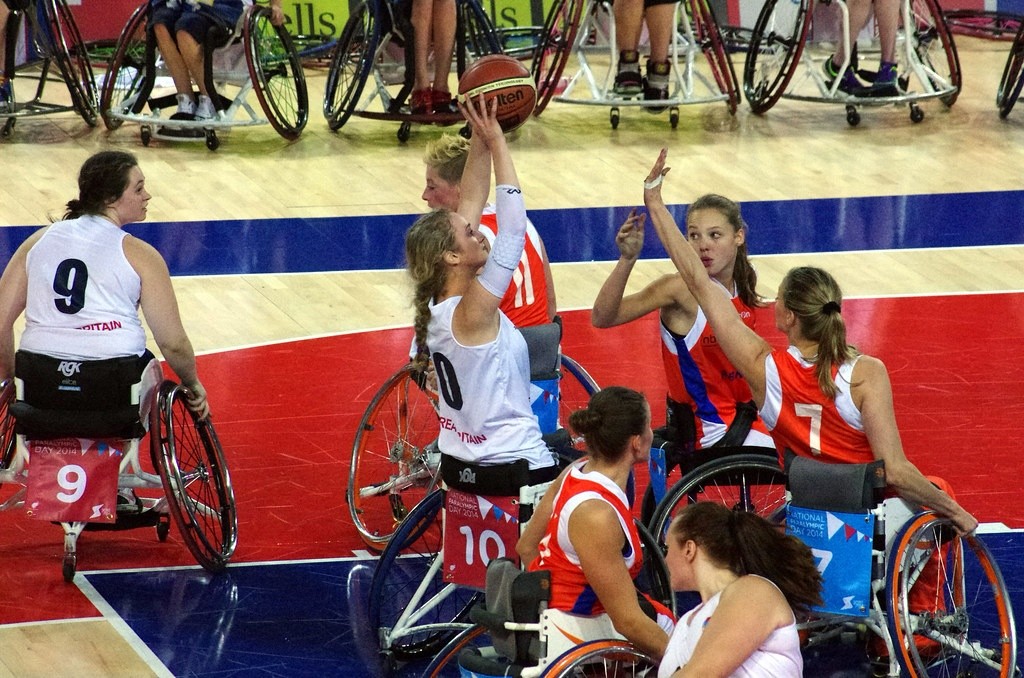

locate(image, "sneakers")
[[644, 60, 670, 113], [823, 54, 869, 98], [613, 49, 643, 94], [871, 62, 898, 97]]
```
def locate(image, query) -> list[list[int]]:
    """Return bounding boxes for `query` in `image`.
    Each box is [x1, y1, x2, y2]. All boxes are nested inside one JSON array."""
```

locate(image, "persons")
[[514, 385, 678, 656], [401, 87, 636, 508], [147, 0, 283, 121], [0, 147, 209, 510], [822, 0, 902, 104], [612, 0, 684, 115], [405, 0, 457, 111], [644, 144, 979, 678], [591, 192, 777, 448], [656, 499, 826, 678]]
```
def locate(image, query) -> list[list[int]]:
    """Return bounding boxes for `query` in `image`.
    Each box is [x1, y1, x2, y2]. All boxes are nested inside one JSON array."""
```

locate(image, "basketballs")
[[458, 53, 538, 133]]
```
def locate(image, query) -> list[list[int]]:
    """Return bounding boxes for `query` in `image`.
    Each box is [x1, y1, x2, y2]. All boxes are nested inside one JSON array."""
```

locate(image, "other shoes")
[[410, 89, 433, 114], [0, 72, 12, 101], [115, 487, 144, 514], [175, 94, 195, 120], [197, 96, 222, 123], [433, 90, 452, 112]]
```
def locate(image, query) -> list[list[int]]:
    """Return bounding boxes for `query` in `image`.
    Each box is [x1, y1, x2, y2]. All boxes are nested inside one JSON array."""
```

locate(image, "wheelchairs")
[[322, 1, 506, 143], [0, 378, 240, 585], [0, 0, 101, 140], [346, 314, 1024, 678], [996, 15, 1024, 121], [100, 0, 309, 152], [528, 0, 743, 130], [743, 0, 963, 128]]
